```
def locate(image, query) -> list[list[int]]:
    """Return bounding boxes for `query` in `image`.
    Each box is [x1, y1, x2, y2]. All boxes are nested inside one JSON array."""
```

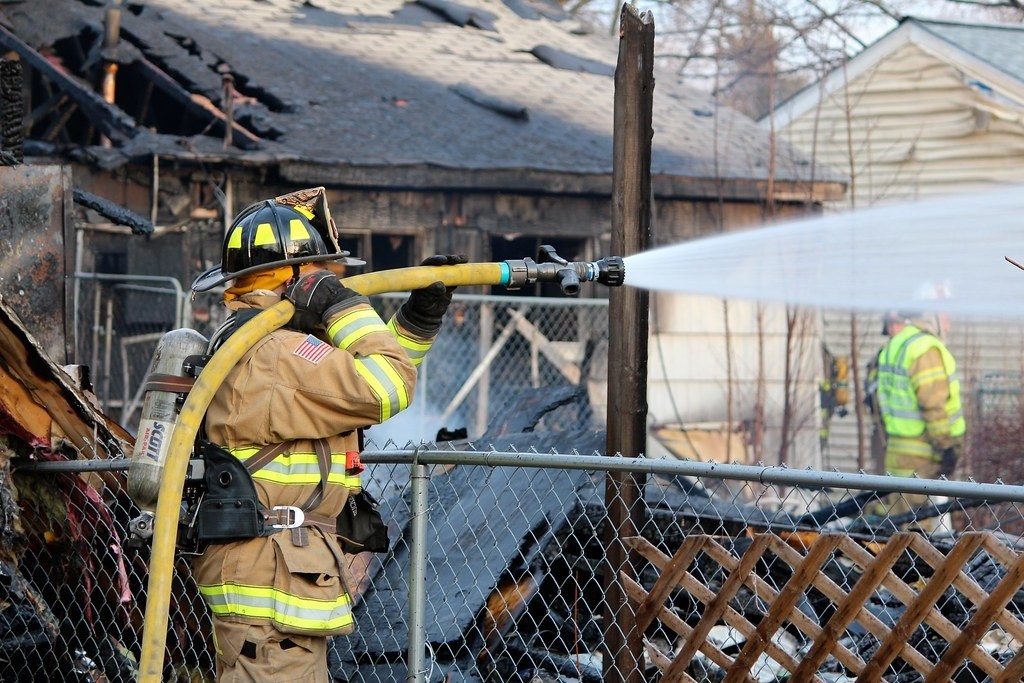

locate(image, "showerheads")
[[522, 244, 626, 297]]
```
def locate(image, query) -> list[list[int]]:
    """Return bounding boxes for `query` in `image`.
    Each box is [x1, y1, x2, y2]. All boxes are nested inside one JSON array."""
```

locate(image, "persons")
[[818, 341, 849, 453], [875, 308, 968, 545], [861, 307, 907, 519], [190, 185, 470, 683]]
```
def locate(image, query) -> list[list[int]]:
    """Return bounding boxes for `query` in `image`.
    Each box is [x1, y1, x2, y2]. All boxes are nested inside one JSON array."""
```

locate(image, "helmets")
[[191, 200, 367, 291]]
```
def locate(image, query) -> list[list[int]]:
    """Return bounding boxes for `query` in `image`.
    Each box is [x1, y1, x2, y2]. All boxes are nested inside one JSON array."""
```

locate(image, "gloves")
[[281, 269, 362, 324], [937, 444, 960, 475], [402, 253, 469, 330]]
